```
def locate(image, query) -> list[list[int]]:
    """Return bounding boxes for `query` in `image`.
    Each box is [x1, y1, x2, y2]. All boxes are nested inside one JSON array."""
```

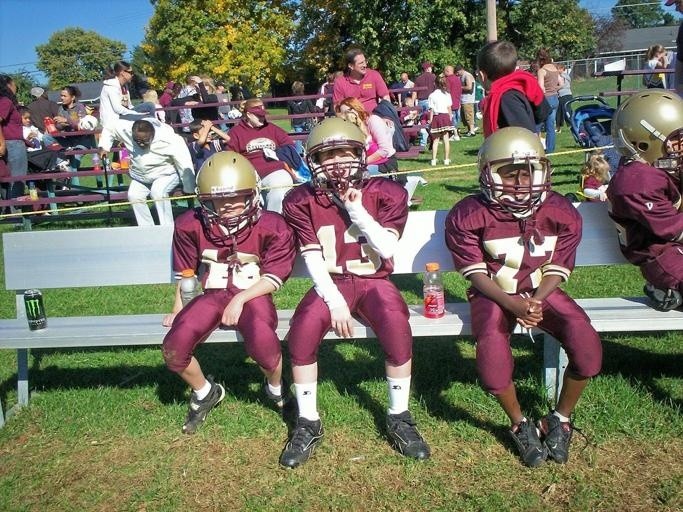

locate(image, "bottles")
[[92, 152, 100, 171], [43, 115, 57, 135], [102, 157, 110, 170], [28, 181, 37, 200], [179, 268, 201, 307], [422, 262, 446, 319]]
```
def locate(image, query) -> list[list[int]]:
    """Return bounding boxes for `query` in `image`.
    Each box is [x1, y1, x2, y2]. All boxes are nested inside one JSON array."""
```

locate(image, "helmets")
[[305, 117, 367, 194], [194, 151, 266, 241], [611, 90, 683, 181], [478, 127, 553, 218]]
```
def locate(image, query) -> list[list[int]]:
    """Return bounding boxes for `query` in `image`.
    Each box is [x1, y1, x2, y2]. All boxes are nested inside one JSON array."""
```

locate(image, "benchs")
[[592, 68, 683, 91], [0, 199, 683, 427], [0, 84, 427, 217], [599, 89, 681, 109]]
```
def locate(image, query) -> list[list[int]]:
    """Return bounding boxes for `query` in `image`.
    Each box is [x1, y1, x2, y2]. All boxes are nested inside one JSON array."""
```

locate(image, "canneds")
[[24, 289, 47, 330]]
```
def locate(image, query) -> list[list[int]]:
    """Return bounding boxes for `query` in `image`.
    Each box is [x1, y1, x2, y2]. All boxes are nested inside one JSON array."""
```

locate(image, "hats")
[[29, 87, 47, 101], [244, 99, 269, 117], [422, 63, 435, 70]]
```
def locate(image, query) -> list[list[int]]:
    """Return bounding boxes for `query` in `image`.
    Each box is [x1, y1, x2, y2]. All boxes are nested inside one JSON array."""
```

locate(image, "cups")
[[119, 159, 128, 170], [31, 127, 38, 138]]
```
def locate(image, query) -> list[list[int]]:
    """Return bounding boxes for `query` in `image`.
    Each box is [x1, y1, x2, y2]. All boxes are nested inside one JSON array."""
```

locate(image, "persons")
[[280, 117, 430, 468], [643, 44, 668, 89], [286, 40, 574, 174], [162, 151, 295, 434], [445, 127, 602, 467], [606, 88, 683, 312], [0, 61, 297, 226]]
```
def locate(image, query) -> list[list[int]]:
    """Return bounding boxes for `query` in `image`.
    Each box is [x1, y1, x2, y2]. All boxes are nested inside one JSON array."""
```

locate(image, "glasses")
[[124, 69, 134, 75]]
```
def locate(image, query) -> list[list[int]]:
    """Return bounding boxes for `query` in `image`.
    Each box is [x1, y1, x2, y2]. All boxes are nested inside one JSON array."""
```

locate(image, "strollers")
[[558, 94, 624, 204]]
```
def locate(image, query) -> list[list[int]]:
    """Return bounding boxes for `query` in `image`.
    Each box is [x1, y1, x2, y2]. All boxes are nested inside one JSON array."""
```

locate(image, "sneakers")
[[385, 409, 431, 463], [642, 282, 683, 310], [449, 135, 461, 141], [444, 159, 452, 166], [536, 411, 574, 464], [507, 415, 548, 468], [464, 131, 476, 137], [431, 159, 439, 167], [182, 378, 225, 435], [262, 375, 299, 423], [280, 414, 324, 470]]
